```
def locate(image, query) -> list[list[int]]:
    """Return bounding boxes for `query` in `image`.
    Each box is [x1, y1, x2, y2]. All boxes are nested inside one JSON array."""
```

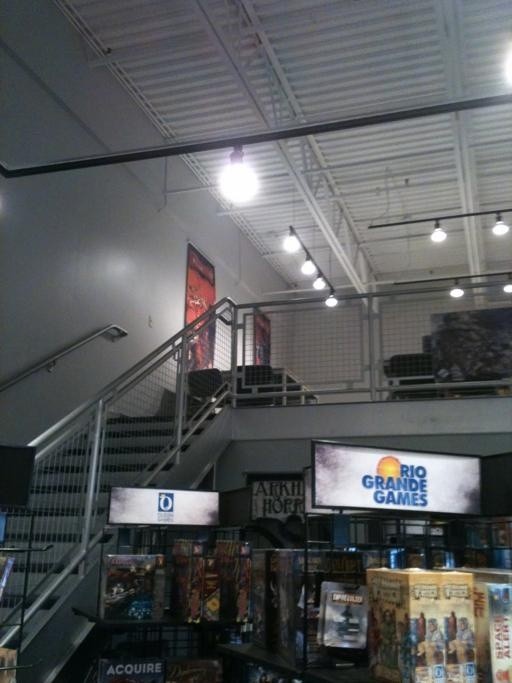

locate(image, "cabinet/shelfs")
[[84, 514, 511, 682]]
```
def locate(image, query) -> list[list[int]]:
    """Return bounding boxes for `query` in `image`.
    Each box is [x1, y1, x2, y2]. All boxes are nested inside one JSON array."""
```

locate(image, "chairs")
[[186, 364, 275, 417]]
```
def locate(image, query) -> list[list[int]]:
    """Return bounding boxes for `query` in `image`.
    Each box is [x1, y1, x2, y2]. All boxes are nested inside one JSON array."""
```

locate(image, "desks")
[[265, 366, 319, 405]]
